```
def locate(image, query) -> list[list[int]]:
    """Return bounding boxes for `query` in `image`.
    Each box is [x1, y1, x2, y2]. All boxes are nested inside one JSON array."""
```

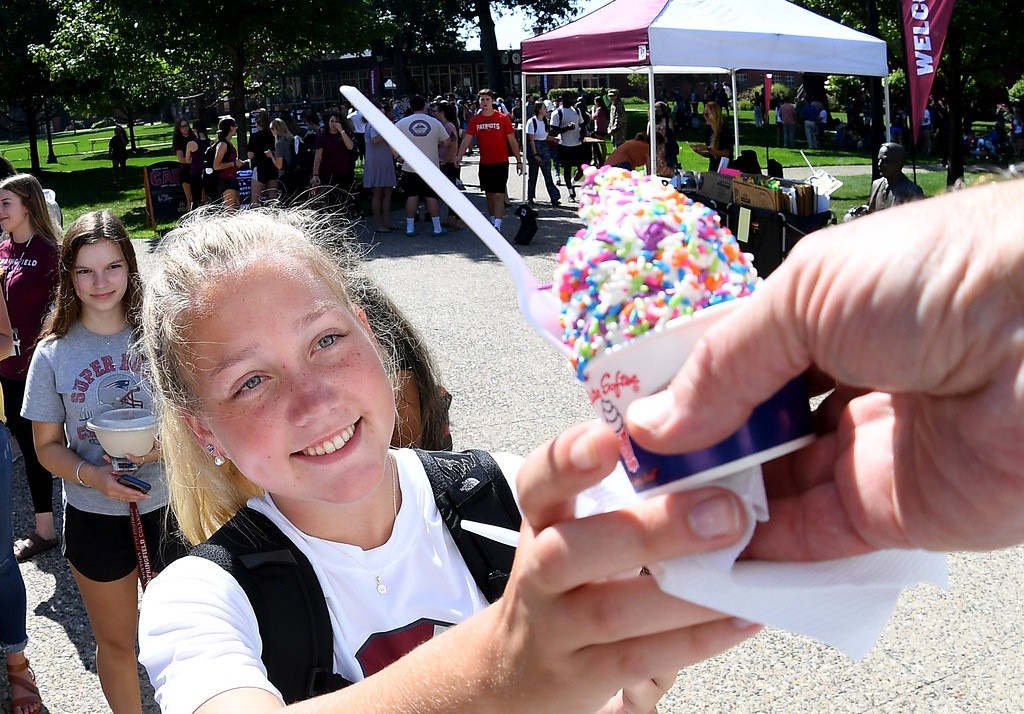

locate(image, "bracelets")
[[517, 162, 522, 164], [76, 461, 91, 488]]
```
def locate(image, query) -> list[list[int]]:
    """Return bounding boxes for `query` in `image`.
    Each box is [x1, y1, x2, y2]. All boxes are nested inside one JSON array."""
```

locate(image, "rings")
[[119, 499, 121, 503], [138, 457, 144, 466]]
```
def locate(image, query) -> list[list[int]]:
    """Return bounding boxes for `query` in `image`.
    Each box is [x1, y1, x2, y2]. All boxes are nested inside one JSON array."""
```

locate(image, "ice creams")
[[555, 163, 764, 380]]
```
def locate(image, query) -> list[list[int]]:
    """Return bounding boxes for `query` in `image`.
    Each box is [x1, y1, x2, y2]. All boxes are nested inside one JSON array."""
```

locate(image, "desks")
[[557, 137, 607, 169]]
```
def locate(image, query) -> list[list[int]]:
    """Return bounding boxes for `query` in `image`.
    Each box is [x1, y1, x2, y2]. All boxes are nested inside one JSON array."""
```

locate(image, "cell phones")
[[117, 475, 151, 494]]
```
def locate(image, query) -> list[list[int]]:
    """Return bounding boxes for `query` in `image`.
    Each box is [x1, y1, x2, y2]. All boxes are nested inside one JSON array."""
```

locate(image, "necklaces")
[[97, 334, 116, 345], [306, 450, 398, 594]]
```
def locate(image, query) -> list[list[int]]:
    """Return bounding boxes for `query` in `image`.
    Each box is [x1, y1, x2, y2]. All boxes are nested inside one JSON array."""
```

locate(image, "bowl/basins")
[[83, 409, 158, 456], [581, 294, 814, 500]]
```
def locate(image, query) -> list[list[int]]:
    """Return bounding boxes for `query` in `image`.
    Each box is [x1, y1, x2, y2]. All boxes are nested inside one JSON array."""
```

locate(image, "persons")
[[345, 277, 453, 451], [131, 201, 763, 714], [750, 88, 1024, 166], [848, 143, 925, 217], [109, 126, 129, 184], [525, 79, 733, 206], [626, 179, 1024, 562], [0, 157, 193, 714], [172, 89, 524, 236]]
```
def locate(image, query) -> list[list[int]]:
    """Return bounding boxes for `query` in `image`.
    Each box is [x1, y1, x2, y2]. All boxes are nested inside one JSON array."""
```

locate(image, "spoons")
[[341, 85, 578, 361]]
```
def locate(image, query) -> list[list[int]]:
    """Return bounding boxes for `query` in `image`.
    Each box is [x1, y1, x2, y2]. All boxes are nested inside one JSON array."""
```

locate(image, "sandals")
[[5, 658, 42, 714], [13, 531, 59, 561]]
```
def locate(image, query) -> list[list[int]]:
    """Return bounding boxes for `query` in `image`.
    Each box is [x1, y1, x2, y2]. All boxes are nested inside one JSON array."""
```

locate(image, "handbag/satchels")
[[423, 385, 453, 453]]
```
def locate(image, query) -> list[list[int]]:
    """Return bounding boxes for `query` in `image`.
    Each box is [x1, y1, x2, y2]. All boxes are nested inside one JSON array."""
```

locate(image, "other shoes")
[[414, 211, 433, 224], [555, 176, 561, 186], [373, 223, 400, 234], [432, 228, 447, 236], [448, 219, 463, 232], [489, 216, 502, 231], [528, 201, 535, 205], [552, 200, 561, 207], [571, 177, 577, 186], [407, 232, 416, 237], [568, 194, 575, 203]]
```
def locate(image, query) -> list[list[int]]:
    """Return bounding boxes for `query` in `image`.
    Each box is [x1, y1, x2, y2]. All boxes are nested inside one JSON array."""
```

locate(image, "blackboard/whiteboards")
[[144, 161, 189, 225]]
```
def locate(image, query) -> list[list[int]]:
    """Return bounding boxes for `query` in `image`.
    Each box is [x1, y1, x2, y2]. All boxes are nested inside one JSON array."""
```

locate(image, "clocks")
[[502, 54, 509, 64], [512, 53, 520, 64]]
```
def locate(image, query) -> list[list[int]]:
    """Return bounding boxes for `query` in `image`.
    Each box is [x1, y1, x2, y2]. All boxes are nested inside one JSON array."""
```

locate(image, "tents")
[[521, 0, 890, 201]]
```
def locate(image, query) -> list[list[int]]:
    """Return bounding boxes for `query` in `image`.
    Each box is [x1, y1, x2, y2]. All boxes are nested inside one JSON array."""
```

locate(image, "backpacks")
[[293, 135, 314, 170], [202, 140, 231, 182]]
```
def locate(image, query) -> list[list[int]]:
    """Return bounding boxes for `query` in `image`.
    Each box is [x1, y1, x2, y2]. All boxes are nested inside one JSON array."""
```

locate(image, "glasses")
[[233, 124, 238, 129]]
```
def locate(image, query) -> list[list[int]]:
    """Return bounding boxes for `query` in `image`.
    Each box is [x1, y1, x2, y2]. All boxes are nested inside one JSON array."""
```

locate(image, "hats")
[[608, 89, 620, 96]]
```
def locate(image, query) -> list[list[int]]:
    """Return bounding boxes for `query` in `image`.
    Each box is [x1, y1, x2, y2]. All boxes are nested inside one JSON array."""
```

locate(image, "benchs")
[[0, 146, 30, 159], [127, 133, 167, 146], [89, 137, 112, 151], [51, 141, 80, 154]]
